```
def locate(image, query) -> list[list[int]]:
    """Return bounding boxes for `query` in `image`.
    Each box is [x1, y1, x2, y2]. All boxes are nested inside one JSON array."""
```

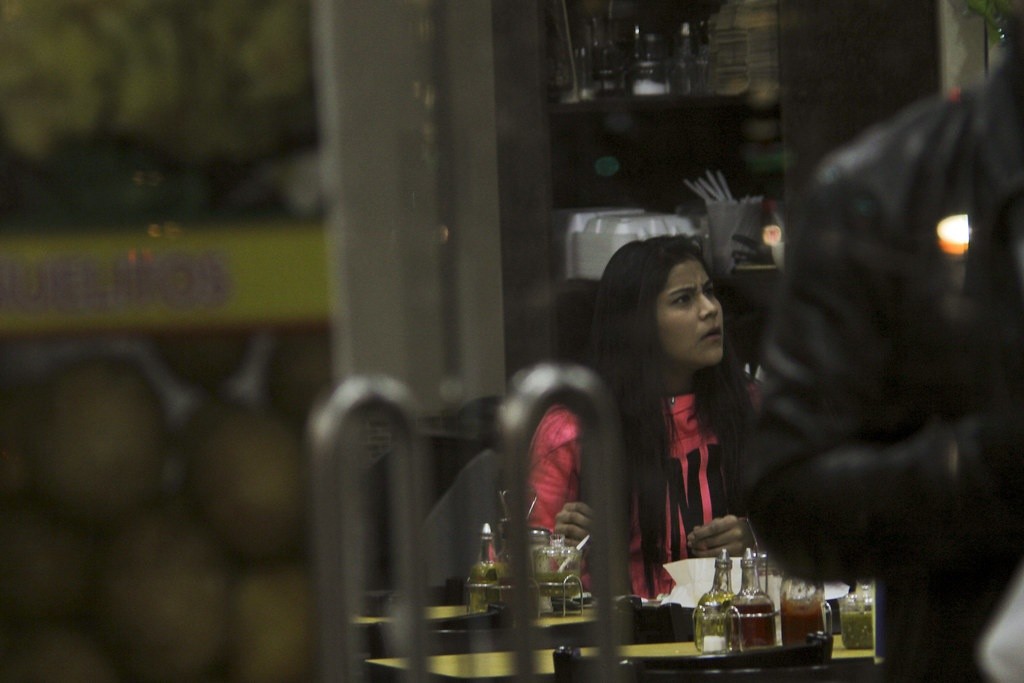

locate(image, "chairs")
[[548, 603, 691, 647], [335, 601, 514, 683], [558, 630, 834, 680]]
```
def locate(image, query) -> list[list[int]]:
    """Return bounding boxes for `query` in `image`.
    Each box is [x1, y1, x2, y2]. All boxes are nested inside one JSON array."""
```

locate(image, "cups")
[[838, 595, 874, 649], [782, 569, 824, 649]]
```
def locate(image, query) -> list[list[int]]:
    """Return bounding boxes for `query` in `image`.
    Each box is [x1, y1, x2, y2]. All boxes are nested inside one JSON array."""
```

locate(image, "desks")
[[362, 634, 873, 683], [350, 596, 659, 644]]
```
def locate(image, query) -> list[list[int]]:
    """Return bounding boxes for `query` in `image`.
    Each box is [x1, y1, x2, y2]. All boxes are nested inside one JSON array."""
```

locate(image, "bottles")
[[732, 560, 777, 651], [532, 531, 551, 611], [693, 559, 736, 654], [467, 538, 500, 612], [539, 533, 581, 593]]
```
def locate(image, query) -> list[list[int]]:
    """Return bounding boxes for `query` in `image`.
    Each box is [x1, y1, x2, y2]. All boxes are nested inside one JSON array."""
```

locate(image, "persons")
[[488, 233, 728, 599], [734, 0, 1022, 683]]
